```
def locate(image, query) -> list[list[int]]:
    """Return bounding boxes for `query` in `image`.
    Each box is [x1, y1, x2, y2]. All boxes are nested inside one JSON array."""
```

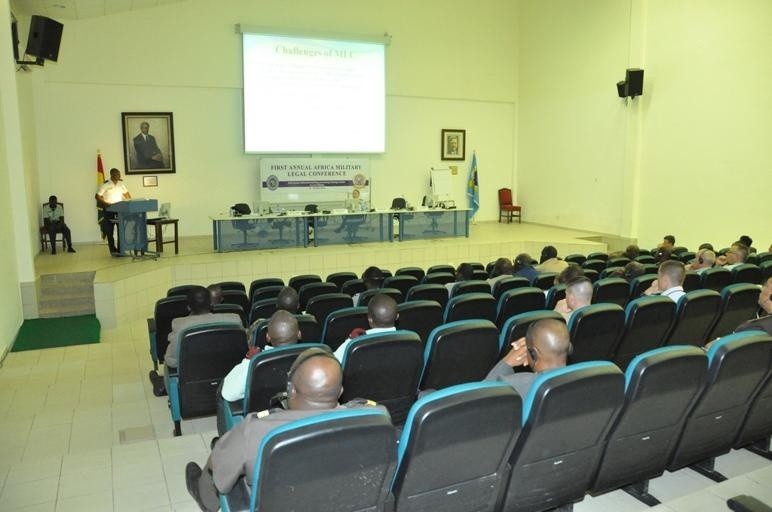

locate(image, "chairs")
[[341, 198, 367, 242], [230, 203, 260, 249], [389, 198, 416, 237], [39, 203, 65, 252], [267, 210, 295, 244], [304, 204, 330, 244], [423, 196, 445, 234], [498, 188, 521, 224]]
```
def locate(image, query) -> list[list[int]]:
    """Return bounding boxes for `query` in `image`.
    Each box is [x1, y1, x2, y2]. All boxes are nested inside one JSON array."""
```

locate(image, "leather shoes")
[[186, 461, 213, 512], [150, 371, 165, 397]]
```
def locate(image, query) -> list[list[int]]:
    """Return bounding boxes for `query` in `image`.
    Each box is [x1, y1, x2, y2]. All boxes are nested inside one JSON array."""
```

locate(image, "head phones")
[[281, 351, 345, 402], [527, 317, 573, 363], [697, 249, 708, 263]]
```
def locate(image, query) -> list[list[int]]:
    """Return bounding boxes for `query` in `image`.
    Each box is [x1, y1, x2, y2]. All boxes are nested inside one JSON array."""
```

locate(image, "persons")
[[445, 244, 593, 402], [210, 310, 302, 448], [185, 346, 391, 512], [446, 134, 461, 157], [42, 195, 77, 256], [148, 286, 245, 396], [207, 284, 224, 306], [131, 121, 166, 170], [248, 286, 316, 342], [351, 266, 384, 307], [333, 189, 370, 233], [607, 235, 772, 316], [333, 293, 399, 364], [94, 167, 132, 258]]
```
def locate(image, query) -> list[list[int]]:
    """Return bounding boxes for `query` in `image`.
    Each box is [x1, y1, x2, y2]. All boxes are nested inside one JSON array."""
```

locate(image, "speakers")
[[616, 68, 644, 99], [25, 14, 64, 64]]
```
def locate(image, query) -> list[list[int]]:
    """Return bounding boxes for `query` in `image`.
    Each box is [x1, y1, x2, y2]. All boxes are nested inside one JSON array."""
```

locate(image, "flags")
[[465, 149, 480, 219], [96, 154, 107, 241]]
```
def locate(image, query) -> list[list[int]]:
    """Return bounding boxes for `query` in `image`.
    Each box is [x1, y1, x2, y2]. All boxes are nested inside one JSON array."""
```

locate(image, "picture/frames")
[[121, 112, 176, 175], [441, 129, 466, 160]]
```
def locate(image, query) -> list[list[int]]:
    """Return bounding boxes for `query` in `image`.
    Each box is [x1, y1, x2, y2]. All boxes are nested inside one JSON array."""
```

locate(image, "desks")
[[110, 217, 179, 255], [209, 208, 473, 252]]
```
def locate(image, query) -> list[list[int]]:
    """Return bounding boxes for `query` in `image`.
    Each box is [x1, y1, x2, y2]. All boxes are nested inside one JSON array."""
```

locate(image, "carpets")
[[11, 314, 100, 353]]
